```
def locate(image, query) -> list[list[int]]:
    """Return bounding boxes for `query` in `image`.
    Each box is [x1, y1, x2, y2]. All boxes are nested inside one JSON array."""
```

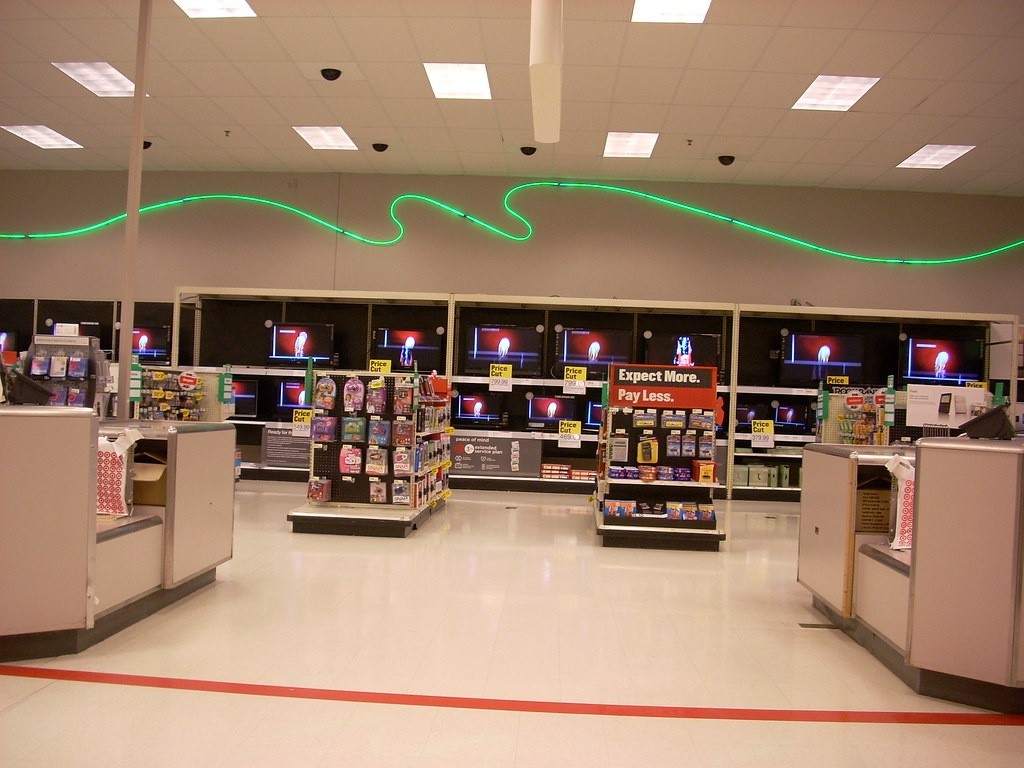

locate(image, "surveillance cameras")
[[321, 69, 341, 81], [719, 156, 734, 165], [143, 142, 151, 148], [373, 144, 388, 152], [521, 147, 536, 155]]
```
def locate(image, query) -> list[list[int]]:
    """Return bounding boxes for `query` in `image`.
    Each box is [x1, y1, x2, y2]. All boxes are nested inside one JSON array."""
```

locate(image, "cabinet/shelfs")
[[596, 407, 717, 531], [307, 372, 451, 509]]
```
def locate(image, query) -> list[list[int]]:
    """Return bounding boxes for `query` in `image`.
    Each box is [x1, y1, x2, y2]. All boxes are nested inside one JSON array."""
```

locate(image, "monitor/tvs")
[[781, 331, 865, 390], [269, 322, 334, 366], [460, 321, 544, 378], [231, 379, 258, 417], [526, 395, 576, 429], [554, 327, 633, 378], [277, 380, 307, 406], [774, 405, 807, 426], [454, 393, 501, 426], [583, 399, 602, 430], [372, 327, 444, 374], [902, 337, 985, 385], [737, 405, 768, 427], [644, 332, 722, 386], [133, 323, 171, 366]]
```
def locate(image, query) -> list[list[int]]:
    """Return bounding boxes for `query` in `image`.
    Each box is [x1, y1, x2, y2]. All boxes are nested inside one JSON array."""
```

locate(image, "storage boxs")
[[132, 451, 168, 507], [691, 458, 719, 483], [733, 464, 790, 488], [855, 476, 891, 534]]
